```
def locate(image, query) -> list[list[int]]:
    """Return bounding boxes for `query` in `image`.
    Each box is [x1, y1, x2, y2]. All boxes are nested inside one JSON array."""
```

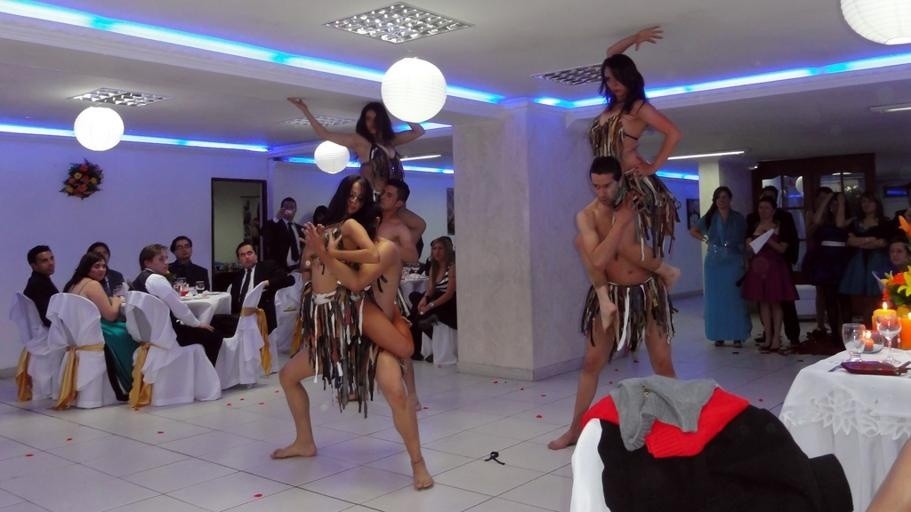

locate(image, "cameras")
[[285, 210, 293, 215]]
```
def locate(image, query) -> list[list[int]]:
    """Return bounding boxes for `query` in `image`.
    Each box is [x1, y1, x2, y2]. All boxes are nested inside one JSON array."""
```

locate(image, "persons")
[[859, 434, 911, 512], [260, 198, 305, 273], [547, 155, 679, 451], [271, 91, 438, 490], [574, 26, 682, 332], [23, 234, 285, 398], [688, 182, 910, 352], [401, 233, 456, 361]]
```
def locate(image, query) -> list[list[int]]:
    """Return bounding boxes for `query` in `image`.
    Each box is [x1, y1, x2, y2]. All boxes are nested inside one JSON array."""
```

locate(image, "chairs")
[[416, 314, 457, 368], [569, 377, 825, 511], [10, 292, 118, 409], [126, 290, 223, 408], [214, 280, 269, 394]]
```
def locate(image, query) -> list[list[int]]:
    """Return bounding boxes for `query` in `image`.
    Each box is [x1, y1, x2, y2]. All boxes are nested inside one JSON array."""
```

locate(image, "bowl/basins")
[[863, 331, 885, 354]]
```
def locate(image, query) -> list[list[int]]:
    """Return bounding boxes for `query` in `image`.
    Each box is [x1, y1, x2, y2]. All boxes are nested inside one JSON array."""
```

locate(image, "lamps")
[[313, 140, 350, 174], [840, 1, 910, 46], [380, 56, 447, 124], [73, 106, 125, 152]]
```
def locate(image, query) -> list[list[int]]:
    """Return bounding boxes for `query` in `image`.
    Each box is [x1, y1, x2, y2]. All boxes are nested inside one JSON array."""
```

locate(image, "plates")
[[841, 361, 896, 374]]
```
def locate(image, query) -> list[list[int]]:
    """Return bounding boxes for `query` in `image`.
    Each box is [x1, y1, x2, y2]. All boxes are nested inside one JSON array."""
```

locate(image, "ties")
[[287, 223, 300, 263], [239, 269, 252, 305]]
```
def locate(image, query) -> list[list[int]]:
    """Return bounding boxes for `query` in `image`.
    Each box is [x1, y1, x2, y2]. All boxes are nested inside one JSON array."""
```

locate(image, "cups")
[[195, 281, 204, 295], [174, 278, 189, 296]]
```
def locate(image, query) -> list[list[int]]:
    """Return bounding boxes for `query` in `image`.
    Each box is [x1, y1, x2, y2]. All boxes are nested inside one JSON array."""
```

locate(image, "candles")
[[873, 309, 898, 329]]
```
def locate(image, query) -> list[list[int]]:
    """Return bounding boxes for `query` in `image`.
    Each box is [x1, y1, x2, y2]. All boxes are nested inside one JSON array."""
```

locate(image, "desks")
[[396, 272, 431, 362], [176, 286, 232, 325], [780, 341, 911, 512]]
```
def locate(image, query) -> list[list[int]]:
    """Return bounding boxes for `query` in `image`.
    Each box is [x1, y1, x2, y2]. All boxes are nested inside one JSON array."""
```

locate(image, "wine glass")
[[403, 262, 420, 280], [876, 316, 904, 365], [841, 323, 867, 362]]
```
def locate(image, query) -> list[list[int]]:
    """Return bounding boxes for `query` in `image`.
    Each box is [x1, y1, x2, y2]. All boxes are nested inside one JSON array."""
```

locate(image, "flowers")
[[871, 214, 911, 305]]
